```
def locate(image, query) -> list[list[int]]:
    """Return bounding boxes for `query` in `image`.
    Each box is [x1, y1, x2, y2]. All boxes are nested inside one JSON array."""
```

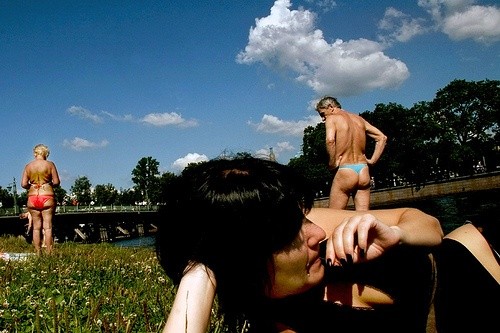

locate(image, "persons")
[[21, 144, 60, 257], [20, 212, 50, 248], [153, 154, 500, 333], [315, 95, 388, 210]]
